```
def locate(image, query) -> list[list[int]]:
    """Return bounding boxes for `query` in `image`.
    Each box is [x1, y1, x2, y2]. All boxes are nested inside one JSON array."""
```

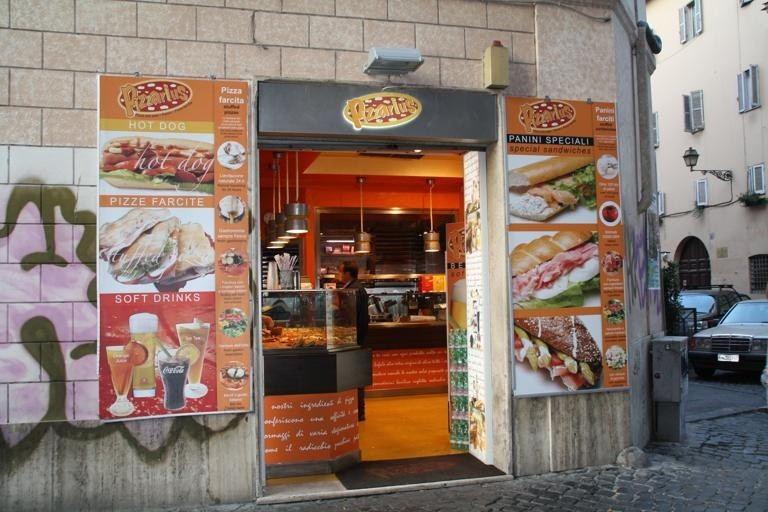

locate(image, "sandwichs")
[[98, 207, 216, 287]]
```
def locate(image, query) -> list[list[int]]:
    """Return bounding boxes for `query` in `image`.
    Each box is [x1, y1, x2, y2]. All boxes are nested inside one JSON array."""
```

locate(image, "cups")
[[104, 312, 210, 417], [266, 262, 300, 291]]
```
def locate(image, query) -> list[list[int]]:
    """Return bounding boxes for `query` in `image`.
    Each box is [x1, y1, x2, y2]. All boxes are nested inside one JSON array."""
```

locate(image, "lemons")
[[175, 343, 201, 367], [124, 340, 148, 365]]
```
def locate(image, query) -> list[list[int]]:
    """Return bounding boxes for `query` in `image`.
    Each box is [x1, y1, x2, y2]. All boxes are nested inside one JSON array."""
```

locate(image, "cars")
[[668, 285, 767, 384]]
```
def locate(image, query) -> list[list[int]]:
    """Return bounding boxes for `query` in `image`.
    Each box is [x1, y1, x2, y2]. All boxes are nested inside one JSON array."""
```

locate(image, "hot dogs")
[[514, 316, 602, 392], [100, 135, 215, 194], [508, 155, 596, 222]]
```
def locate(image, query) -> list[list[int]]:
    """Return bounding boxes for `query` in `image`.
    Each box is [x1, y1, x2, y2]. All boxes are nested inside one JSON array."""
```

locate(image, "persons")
[[336, 259, 370, 422]]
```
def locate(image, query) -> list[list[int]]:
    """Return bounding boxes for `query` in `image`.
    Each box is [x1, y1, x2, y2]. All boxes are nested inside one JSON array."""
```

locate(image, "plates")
[[596, 155, 622, 227], [215, 142, 250, 391]]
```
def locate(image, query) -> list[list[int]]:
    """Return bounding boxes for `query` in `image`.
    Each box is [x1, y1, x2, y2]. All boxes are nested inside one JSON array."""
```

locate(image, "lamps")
[[264, 150, 309, 249], [362, 45, 424, 92], [423, 178, 441, 253], [353, 175, 372, 255]]
